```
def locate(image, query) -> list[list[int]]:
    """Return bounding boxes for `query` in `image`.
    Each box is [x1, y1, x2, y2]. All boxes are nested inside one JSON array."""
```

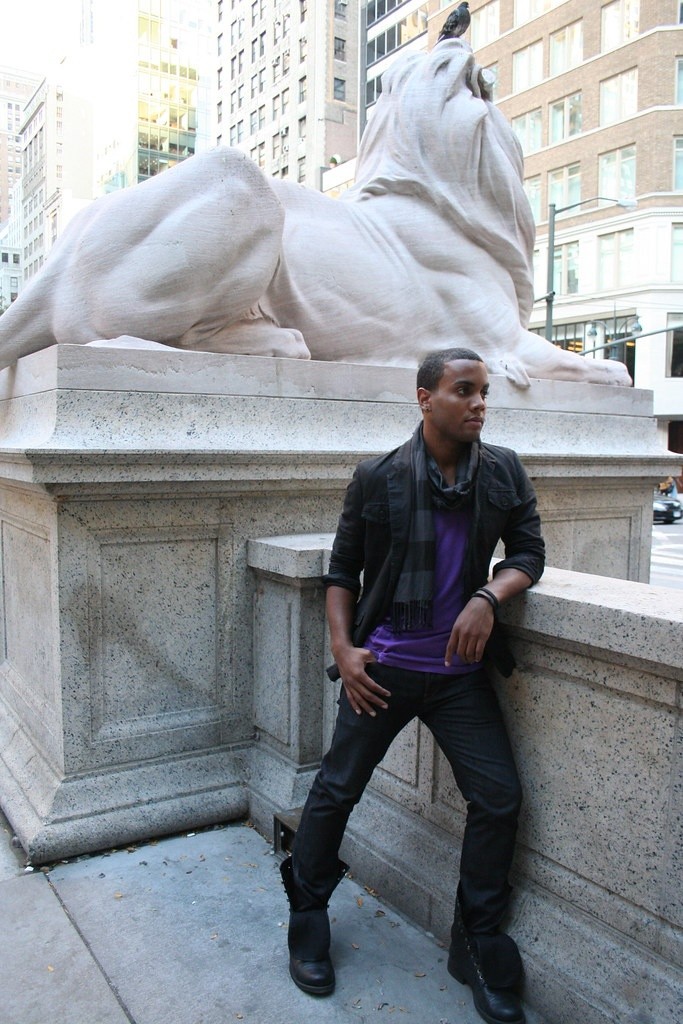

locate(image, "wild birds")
[[433, 1, 471, 46]]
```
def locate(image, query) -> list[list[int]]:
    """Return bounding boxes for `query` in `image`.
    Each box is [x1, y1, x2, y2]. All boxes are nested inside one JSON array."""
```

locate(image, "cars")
[[653, 493, 683, 526]]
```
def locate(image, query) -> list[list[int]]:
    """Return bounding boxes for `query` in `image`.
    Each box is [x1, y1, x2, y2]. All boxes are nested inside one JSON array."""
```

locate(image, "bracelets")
[[470, 587, 500, 615]]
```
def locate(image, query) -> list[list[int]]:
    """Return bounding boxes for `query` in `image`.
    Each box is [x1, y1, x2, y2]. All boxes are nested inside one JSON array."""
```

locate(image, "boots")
[[278, 855, 350, 995], [447, 883, 526, 1024]]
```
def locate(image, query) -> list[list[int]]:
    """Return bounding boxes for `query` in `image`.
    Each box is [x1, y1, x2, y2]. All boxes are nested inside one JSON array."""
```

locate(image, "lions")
[[0, 38, 633, 385]]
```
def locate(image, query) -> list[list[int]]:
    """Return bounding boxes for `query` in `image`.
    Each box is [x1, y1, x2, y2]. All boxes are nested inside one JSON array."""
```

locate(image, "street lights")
[[545, 196, 640, 341], [588, 313, 642, 361]]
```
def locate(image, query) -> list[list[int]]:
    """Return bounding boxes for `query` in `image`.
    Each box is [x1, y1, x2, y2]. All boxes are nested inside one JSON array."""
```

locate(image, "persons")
[[658, 477, 678, 500], [280, 347, 547, 1024]]
